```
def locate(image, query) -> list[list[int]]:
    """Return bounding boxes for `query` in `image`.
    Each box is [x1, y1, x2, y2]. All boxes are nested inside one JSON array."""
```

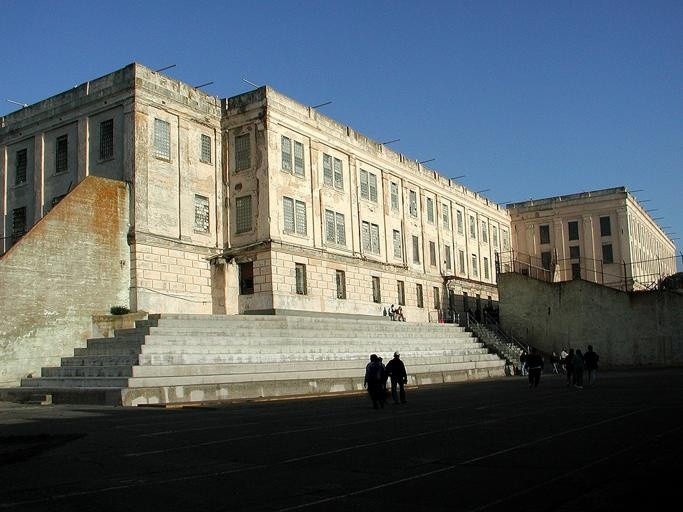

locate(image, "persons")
[[446, 303, 499, 325], [395, 305, 405, 321], [378, 356, 388, 404], [519, 344, 600, 391], [388, 304, 396, 321], [385, 351, 409, 405], [363, 353, 385, 409]]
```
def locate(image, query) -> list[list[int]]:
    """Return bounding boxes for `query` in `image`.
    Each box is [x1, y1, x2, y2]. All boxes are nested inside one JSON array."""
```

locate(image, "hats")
[[394, 351, 400, 357]]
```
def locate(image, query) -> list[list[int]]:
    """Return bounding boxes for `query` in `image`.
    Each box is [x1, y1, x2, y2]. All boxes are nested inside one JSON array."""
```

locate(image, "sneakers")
[[373, 401, 407, 409], [566, 382, 583, 389]]
